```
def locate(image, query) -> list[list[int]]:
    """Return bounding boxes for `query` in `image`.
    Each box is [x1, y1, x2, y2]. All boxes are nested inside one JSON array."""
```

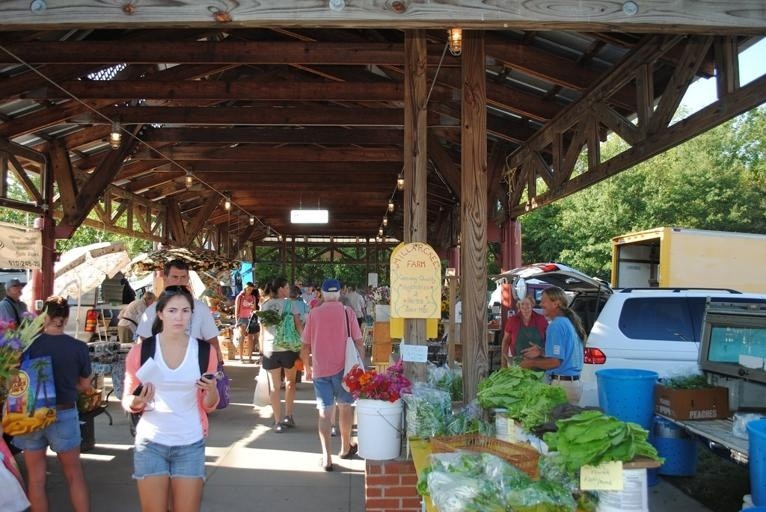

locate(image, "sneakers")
[[272, 424, 282, 433], [281, 416, 294, 426]]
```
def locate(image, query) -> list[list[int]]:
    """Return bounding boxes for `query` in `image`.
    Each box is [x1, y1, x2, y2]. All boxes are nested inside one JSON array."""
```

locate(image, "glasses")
[[164, 286, 189, 296]]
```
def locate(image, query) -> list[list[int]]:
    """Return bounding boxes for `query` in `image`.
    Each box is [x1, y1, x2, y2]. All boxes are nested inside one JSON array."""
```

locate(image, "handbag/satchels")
[[272, 315, 302, 352], [245, 313, 259, 334], [340, 337, 365, 393], [1, 348, 57, 437], [215, 362, 230, 409], [232, 326, 241, 347]]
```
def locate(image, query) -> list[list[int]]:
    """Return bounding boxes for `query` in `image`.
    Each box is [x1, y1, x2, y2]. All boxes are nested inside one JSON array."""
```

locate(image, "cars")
[[489, 278, 577, 315]]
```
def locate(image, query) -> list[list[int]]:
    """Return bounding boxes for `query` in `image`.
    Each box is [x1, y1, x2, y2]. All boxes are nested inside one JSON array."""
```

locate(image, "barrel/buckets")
[[746, 419, 766, 505], [596, 369, 659, 440], [355, 398, 403, 461], [652, 414, 698, 476]]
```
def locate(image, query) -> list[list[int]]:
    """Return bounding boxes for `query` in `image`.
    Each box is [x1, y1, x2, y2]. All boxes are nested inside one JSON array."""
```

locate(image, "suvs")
[[488, 263, 766, 408]]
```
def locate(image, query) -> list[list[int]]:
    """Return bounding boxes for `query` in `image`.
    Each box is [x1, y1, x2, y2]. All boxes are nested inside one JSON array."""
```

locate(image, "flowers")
[[343, 355, 413, 403], [0, 305, 49, 421]]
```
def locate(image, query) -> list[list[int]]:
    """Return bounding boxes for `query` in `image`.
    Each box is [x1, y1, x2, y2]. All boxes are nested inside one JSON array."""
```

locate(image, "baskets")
[[430, 435, 540, 482]]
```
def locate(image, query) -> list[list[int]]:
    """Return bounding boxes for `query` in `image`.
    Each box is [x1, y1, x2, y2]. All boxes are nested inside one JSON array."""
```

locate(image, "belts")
[[55, 403, 74, 410], [551, 376, 579, 380]]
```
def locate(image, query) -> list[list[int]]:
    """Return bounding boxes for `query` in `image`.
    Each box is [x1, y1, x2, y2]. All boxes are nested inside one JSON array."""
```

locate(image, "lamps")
[[447, 28, 462, 56], [110, 121, 122, 150], [388, 199, 395, 212], [185, 171, 193, 188], [397, 173, 404, 190]]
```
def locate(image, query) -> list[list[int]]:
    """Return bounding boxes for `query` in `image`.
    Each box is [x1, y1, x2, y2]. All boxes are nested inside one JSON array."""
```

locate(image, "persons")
[[1, 259, 232, 512], [231, 274, 375, 475], [518, 286, 587, 408], [500, 293, 547, 378]]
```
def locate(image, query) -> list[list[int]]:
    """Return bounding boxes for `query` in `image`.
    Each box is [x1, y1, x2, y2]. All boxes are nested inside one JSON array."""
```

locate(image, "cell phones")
[[131, 385, 148, 398], [196, 373, 214, 393]]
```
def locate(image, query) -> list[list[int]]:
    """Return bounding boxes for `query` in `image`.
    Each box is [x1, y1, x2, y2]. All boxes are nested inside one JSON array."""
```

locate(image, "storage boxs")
[[656, 384, 729, 420]]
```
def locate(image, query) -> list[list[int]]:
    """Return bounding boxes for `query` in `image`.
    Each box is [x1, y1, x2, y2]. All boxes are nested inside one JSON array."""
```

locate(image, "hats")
[[4, 279, 26, 288], [321, 280, 340, 292]]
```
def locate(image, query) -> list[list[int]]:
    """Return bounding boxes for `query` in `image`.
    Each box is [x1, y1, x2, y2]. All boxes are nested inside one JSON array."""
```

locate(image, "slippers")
[[338, 442, 356, 459], [318, 458, 332, 472]]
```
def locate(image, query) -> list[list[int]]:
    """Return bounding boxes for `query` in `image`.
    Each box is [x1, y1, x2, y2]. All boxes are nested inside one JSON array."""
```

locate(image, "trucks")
[[608, 226, 766, 293]]
